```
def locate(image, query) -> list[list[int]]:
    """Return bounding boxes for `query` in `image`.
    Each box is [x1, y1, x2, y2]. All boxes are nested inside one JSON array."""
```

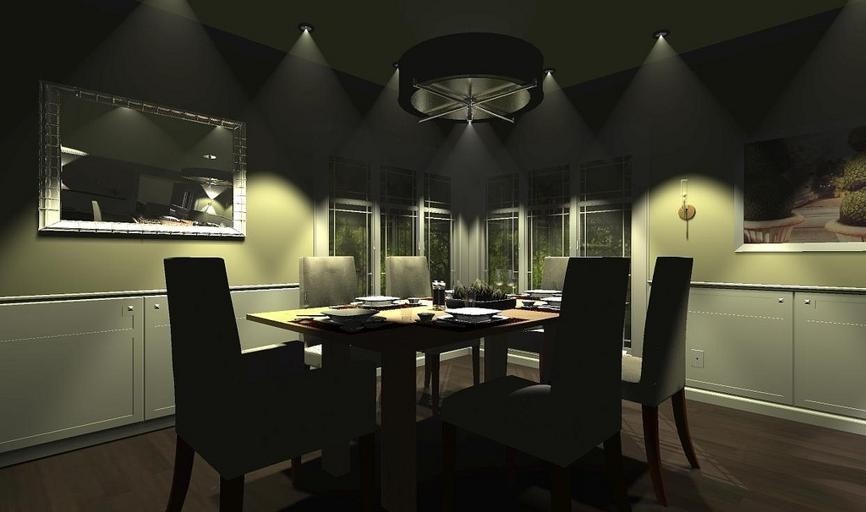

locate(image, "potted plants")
[[743, 154, 865, 242]]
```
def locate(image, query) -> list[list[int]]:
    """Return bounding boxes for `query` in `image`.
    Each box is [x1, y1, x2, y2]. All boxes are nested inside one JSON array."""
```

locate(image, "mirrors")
[[33, 78, 246, 241]]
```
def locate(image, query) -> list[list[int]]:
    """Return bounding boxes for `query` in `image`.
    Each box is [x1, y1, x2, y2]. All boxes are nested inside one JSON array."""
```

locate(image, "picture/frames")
[[732, 116, 865, 253]]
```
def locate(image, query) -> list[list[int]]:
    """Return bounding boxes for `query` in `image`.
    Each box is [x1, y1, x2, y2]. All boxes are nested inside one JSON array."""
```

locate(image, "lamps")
[[396, 32, 545, 127], [677, 176, 695, 221]]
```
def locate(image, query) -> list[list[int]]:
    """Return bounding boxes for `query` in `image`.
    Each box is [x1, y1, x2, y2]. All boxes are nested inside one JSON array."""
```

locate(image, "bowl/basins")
[[321, 308, 380, 323], [355, 295, 401, 305], [542, 297, 562, 306], [444, 307, 501, 321]]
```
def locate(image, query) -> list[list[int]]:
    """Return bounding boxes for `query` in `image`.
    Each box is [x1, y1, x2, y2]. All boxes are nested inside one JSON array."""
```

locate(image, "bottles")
[[432, 281, 446, 310]]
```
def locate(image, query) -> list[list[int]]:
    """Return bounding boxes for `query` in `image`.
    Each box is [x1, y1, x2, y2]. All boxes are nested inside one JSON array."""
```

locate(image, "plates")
[[352, 302, 405, 307], [312, 316, 390, 327], [437, 314, 510, 324]]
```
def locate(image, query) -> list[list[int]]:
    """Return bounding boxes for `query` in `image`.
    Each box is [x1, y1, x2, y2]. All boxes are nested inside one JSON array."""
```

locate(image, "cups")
[[408, 297, 419, 304], [417, 313, 436, 320], [521, 300, 535, 306]]
